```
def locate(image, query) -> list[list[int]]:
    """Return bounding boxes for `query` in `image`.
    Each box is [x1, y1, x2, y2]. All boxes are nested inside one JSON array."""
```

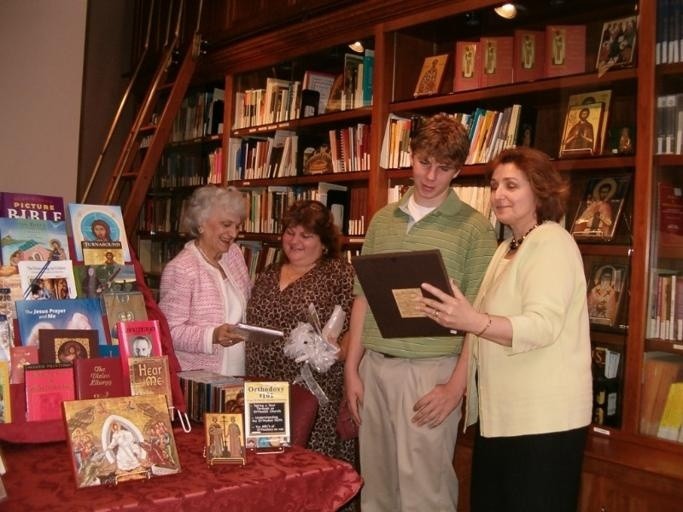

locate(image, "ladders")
[[102, 37, 200, 242]]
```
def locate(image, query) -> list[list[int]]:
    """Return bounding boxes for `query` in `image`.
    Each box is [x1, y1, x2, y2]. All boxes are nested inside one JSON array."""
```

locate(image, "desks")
[[0, 408, 366, 512]]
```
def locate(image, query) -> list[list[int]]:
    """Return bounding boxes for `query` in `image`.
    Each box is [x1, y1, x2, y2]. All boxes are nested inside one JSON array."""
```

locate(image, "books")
[[204, 412, 247, 467], [61, 395, 183, 491]]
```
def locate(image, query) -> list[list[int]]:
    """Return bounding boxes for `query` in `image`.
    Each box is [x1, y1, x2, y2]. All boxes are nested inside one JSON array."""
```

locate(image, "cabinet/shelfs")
[[133, 44, 231, 299], [452, 444, 470, 512], [638, 1, 682, 452], [378, 1, 640, 431], [232, 3, 373, 289], [583, 456, 681, 511]]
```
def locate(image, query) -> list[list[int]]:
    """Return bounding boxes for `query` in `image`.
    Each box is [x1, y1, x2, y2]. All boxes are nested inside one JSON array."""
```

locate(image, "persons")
[[240, 201, 360, 512], [344, 117, 497, 511], [412, 146, 594, 510], [73, 421, 176, 486], [208, 417, 242, 458]]
